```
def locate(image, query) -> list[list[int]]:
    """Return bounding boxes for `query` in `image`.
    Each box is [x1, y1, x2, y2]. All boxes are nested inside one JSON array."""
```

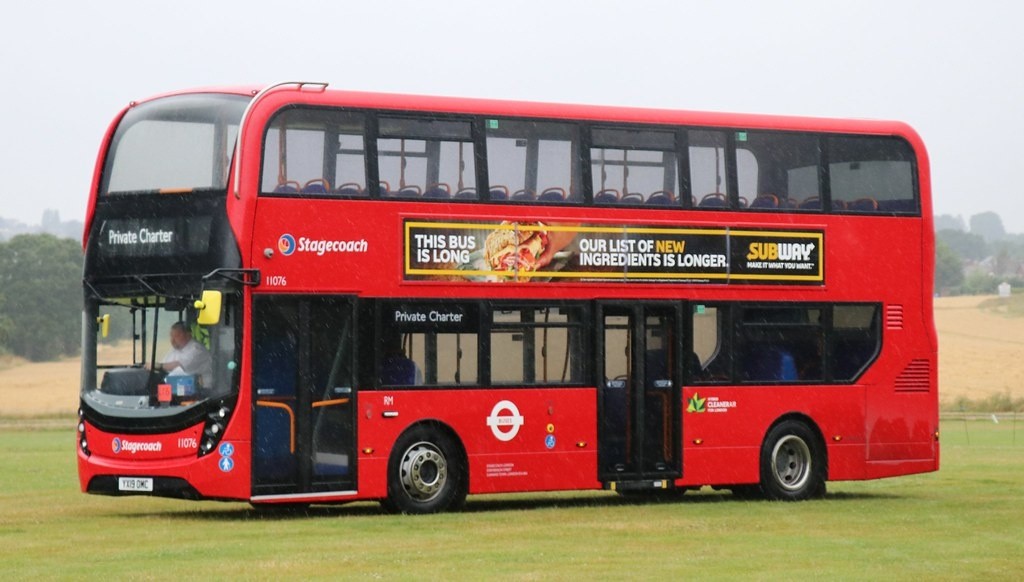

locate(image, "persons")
[[154, 321, 216, 397]]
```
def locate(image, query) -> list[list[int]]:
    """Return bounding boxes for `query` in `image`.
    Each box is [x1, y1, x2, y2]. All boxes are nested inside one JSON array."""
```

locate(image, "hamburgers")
[[483, 220, 547, 282]]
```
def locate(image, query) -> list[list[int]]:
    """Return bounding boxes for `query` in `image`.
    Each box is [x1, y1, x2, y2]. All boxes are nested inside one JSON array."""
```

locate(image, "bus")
[[77, 81, 940, 516]]
